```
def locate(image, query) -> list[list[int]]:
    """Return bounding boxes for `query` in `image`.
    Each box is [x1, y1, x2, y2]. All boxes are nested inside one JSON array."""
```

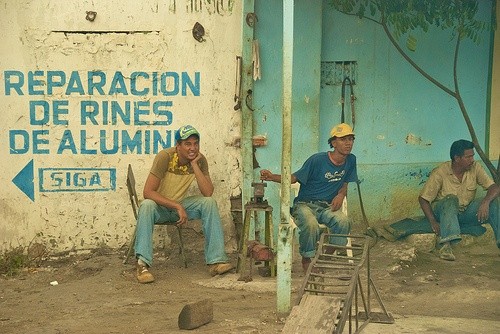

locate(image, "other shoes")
[[210, 263, 233, 276], [440, 246, 456, 260], [137, 264, 155, 283]]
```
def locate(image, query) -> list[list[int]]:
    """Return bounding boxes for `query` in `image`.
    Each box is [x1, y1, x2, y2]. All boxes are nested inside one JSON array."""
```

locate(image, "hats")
[[175, 125, 199, 140], [331, 123, 355, 137]]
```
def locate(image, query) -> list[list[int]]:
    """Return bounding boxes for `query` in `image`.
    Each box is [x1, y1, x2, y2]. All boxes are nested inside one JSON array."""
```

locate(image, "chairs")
[[123, 164, 188, 268]]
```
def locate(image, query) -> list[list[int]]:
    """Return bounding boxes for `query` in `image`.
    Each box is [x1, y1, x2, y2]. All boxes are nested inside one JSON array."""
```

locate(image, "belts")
[[341, 78, 355, 123], [308, 202, 328, 208]]
